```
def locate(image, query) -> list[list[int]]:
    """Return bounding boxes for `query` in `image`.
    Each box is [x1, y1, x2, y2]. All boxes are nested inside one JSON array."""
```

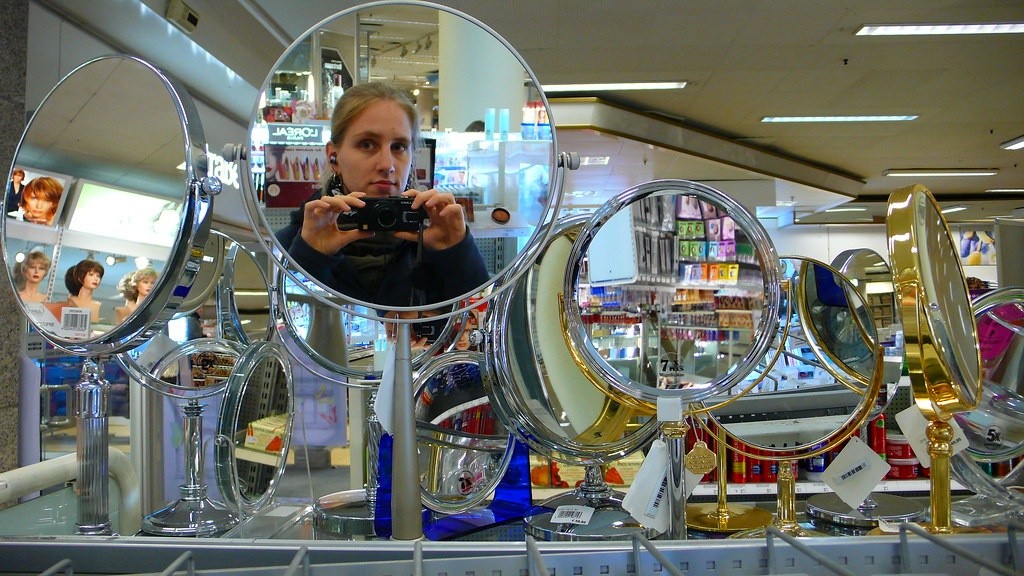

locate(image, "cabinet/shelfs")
[[586, 316, 659, 387], [661, 289, 758, 332]]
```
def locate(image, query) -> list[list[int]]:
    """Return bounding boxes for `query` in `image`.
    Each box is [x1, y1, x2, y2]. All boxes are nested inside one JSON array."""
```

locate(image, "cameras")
[[356, 195, 423, 232]]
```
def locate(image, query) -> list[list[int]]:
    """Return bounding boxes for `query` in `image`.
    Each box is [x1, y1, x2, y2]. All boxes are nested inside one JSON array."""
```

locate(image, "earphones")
[[330, 152, 337, 165]]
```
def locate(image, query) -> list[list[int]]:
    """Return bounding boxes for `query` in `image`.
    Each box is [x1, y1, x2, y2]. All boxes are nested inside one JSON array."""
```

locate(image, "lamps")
[[369, 29, 439, 67], [880, 132, 1024, 215], [15, 239, 155, 272]]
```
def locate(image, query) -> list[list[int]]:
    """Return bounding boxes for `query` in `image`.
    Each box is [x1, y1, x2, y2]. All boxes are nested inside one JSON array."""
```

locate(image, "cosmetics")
[[440, 403, 496, 435], [244, 412, 289, 455], [686, 408, 1024, 482], [529, 450, 645, 489]]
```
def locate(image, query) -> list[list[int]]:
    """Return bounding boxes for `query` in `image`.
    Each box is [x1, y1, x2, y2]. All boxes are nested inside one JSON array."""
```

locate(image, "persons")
[[6, 169, 64, 226], [276, 82, 491, 307], [265, 145, 285, 182], [449, 310, 478, 350], [115, 270, 157, 323], [19, 251, 52, 301], [377, 306, 446, 351], [188, 307, 215, 339], [66, 260, 105, 321]]
